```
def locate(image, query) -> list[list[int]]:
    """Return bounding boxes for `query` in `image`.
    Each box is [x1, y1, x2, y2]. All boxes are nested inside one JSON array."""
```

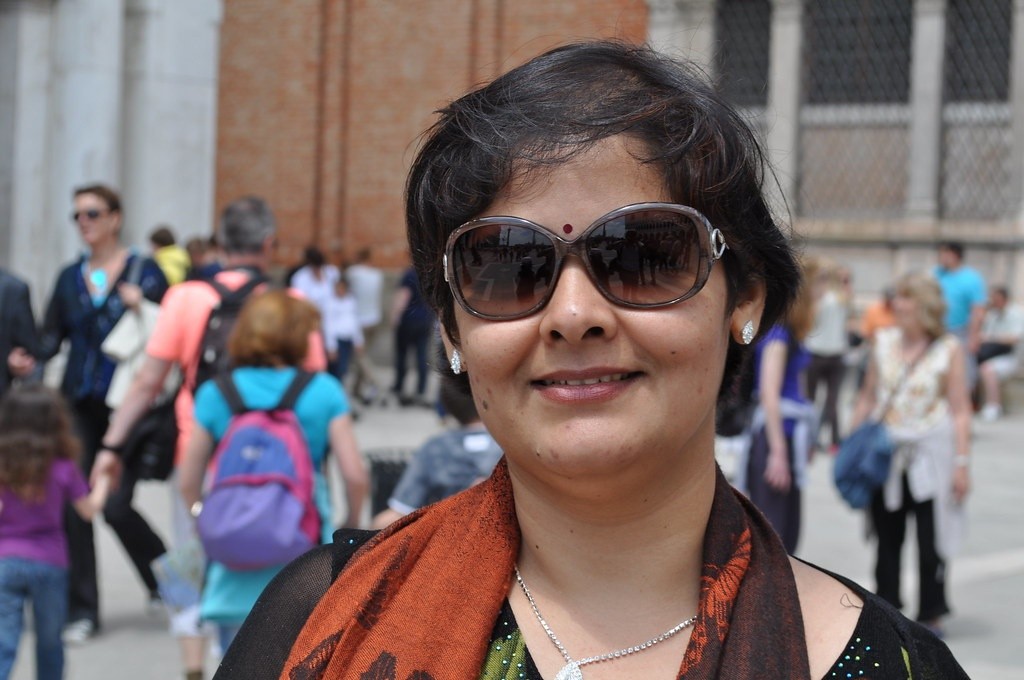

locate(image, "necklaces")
[[515, 564, 702, 679]]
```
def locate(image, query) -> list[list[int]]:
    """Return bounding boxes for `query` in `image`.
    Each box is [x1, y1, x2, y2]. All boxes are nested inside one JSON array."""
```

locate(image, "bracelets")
[[98, 442, 124, 454], [952, 454, 970, 467]]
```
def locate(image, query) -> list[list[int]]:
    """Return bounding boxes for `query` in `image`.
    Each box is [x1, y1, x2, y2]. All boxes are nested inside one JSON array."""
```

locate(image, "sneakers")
[[61, 620, 97, 643], [979, 403, 1002, 421]]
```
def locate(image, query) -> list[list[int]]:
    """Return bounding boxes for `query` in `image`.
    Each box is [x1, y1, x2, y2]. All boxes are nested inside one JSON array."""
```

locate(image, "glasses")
[[440, 201, 729, 323], [74, 208, 103, 220]]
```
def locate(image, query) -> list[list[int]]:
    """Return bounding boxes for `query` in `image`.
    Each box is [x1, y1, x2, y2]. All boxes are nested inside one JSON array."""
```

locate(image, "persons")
[[0, 184, 504, 680], [743, 239, 1024, 638], [210, 35, 970, 680]]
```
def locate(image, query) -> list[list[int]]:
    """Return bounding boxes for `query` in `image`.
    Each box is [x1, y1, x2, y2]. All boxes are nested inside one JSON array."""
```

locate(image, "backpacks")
[[192, 367, 326, 574], [192, 280, 265, 392]]
